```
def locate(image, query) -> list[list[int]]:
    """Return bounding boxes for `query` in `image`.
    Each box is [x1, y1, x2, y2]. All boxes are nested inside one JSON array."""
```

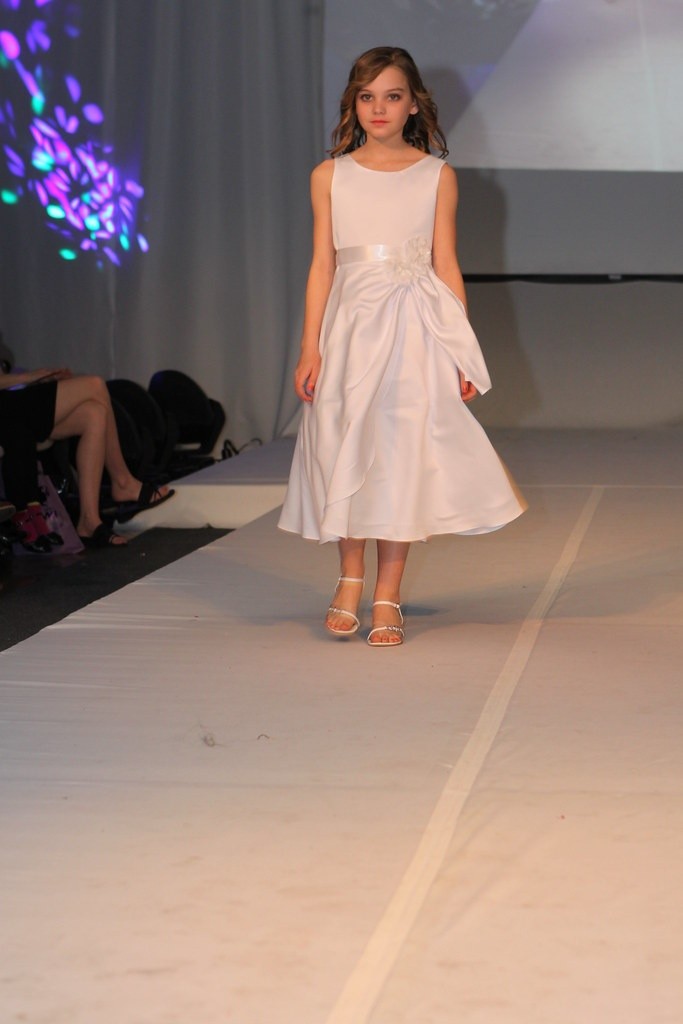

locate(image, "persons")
[[0, 357, 175, 544], [276, 46, 526, 646]]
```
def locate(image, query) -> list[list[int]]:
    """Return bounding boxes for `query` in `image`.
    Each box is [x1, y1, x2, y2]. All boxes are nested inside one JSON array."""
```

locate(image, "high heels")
[[116, 480, 176, 523], [78, 524, 129, 549]]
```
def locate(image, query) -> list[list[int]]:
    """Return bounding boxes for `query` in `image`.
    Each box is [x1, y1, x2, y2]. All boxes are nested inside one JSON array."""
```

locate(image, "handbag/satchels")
[[12, 476, 85, 557]]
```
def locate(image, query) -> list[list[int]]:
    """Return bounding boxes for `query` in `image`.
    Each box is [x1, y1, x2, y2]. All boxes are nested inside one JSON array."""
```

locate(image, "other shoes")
[[13, 518, 53, 554], [31, 513, 64, 546]]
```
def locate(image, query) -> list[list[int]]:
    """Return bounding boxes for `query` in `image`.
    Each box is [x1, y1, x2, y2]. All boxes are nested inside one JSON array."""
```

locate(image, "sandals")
[[367, 600, 404, 647], [325, 574, 366, 636]]
